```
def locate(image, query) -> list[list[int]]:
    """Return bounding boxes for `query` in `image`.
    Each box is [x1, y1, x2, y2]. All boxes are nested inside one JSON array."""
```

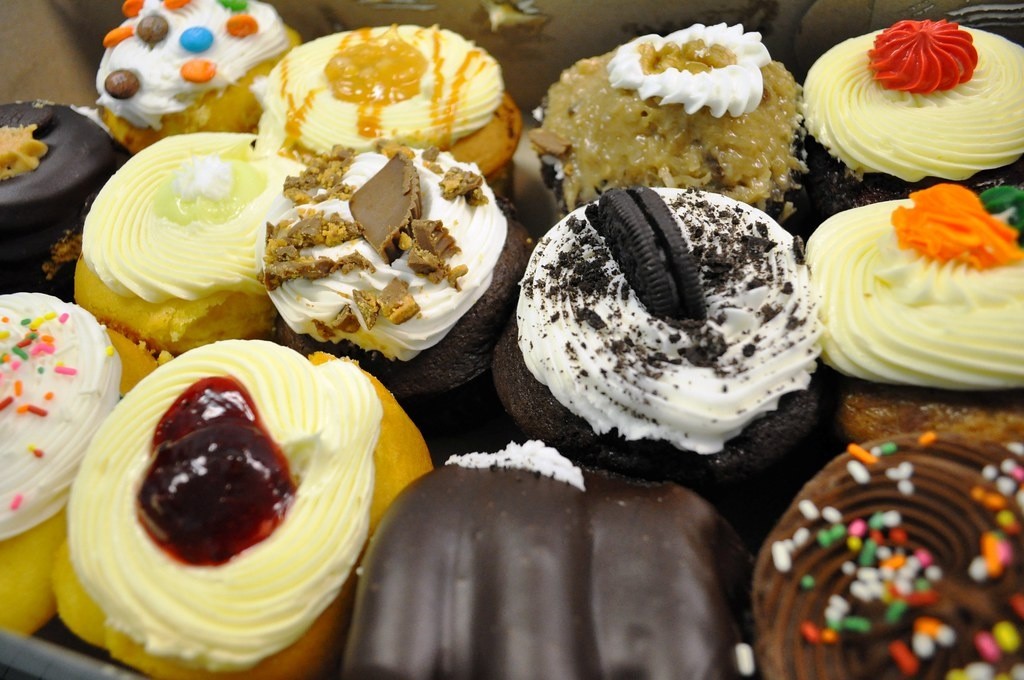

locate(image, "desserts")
[[0, 0, 1024, 680]]
